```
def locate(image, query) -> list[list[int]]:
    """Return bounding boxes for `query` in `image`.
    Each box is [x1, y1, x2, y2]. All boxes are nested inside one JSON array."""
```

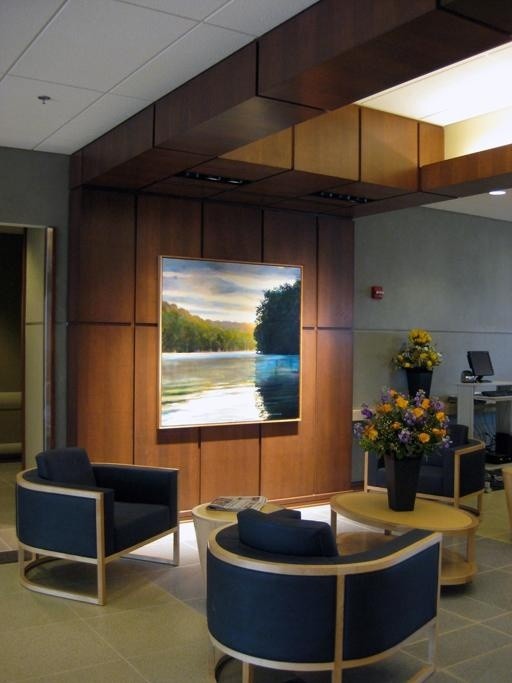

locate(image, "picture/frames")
[[156, 254, 304, 429]]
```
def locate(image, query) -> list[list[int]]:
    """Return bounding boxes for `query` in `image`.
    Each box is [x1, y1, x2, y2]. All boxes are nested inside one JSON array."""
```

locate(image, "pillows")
[[450, 424, 467, 445], [36, 446, 98, 488]]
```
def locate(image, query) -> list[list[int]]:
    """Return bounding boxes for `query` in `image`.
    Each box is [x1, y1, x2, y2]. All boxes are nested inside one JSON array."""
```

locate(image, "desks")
[[458, 379, 512, 443]]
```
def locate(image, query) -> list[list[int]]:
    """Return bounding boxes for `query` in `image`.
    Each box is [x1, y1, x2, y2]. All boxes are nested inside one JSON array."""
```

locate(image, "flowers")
[[352, 385, 453, 466]]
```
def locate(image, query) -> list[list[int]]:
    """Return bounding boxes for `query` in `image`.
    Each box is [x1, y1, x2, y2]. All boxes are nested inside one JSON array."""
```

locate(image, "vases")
[[385, 451, 419, 510], [407, 367, 432, 399]]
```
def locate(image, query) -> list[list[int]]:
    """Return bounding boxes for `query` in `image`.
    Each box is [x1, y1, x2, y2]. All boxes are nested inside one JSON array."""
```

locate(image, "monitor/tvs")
[[467, 351, 494, 383]]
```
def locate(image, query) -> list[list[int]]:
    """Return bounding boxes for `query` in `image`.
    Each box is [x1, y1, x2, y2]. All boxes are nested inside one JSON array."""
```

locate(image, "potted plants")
[[388, 328, 442, 370]]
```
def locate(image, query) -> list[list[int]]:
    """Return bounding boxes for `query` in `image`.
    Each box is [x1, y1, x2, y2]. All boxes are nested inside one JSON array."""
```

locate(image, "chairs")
[[363, 439, 485, 513]]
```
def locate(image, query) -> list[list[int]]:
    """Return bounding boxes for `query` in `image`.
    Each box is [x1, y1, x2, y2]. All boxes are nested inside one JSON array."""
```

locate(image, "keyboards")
[[481, 390, 512, 397]]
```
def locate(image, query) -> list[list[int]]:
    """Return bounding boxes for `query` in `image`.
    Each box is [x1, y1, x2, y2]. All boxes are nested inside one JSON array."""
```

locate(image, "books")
[[207, 495, 266, 511]]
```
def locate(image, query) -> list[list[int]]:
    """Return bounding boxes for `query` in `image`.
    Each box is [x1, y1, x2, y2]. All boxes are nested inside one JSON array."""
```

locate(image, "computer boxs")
[[494, 432, 512, 465]]
